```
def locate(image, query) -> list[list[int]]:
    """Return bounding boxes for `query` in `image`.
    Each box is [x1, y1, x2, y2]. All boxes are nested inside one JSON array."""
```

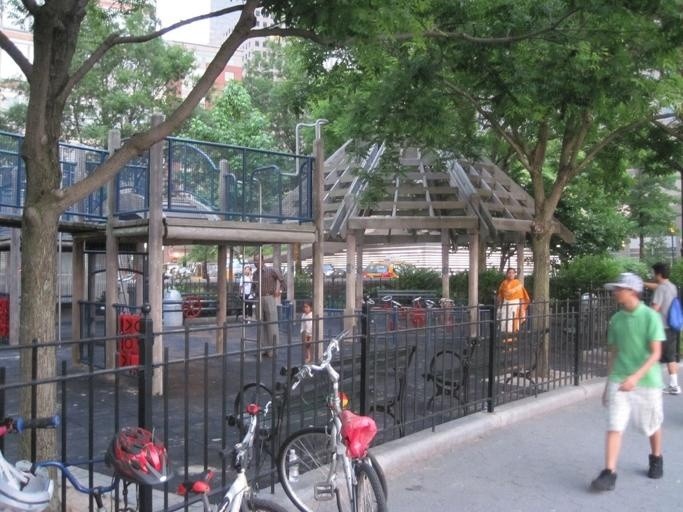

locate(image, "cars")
[[163, 257, 258, 278], [363, 260, 403, 279], [304, 264, 345, 279]]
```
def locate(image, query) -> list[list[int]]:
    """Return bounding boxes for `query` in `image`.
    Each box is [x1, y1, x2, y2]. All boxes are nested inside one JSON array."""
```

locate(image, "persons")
[[643, 262, 681, 395], [299, 300, 312, 363], [496, 268, 531, 344], [250, 253, 284, 358], [591, 272, 667, 491], [239, 266, 252, 324]]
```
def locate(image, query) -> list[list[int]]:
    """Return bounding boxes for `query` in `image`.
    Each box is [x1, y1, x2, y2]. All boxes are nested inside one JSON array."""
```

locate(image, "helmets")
[[106, 426, 174, 487]]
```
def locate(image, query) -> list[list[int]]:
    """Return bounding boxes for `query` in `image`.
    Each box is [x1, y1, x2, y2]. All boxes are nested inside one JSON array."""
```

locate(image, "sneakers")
[[648, 452, 664, 479], [589, 469, 617, 491], [662, 384, 682, 395]]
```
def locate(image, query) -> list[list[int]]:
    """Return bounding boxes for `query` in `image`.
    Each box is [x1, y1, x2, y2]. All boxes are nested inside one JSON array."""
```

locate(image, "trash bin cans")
[[579, 293, 598, 336], [162, 289, 184, 327]]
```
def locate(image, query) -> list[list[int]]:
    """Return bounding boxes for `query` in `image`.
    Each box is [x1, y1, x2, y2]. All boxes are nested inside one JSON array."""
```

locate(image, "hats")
[[603, 272, 644, 292]]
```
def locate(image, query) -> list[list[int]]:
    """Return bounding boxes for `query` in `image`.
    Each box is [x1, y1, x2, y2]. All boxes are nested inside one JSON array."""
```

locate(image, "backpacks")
[[667, 297, 683, 332]]
